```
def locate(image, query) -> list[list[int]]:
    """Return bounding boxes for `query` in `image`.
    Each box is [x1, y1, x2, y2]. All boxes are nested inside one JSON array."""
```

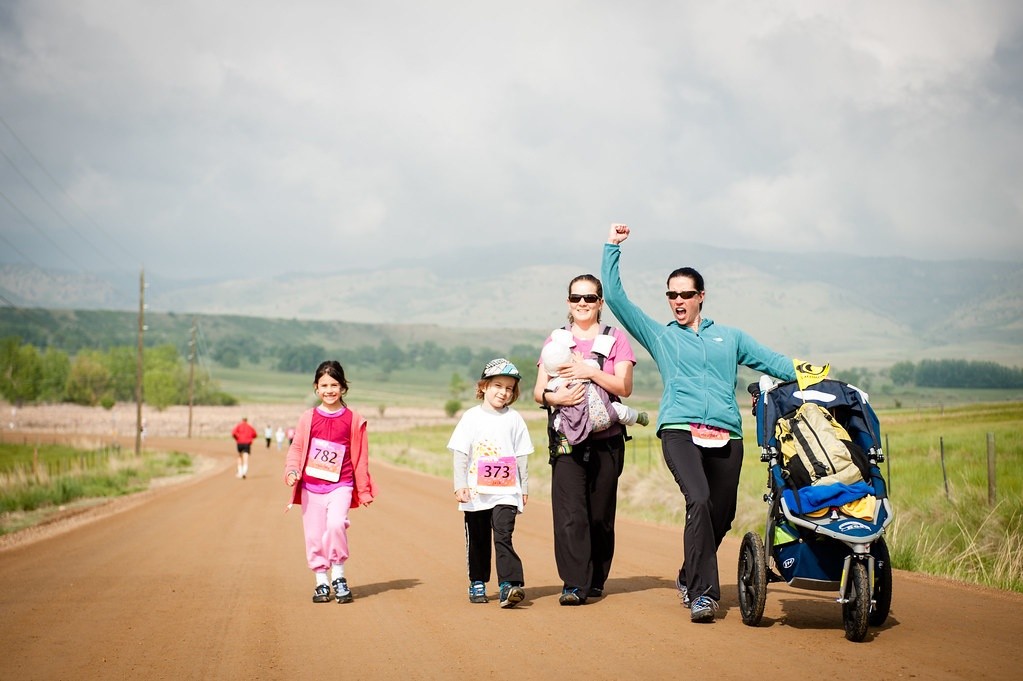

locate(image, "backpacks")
[[775, 403, 865, 518]]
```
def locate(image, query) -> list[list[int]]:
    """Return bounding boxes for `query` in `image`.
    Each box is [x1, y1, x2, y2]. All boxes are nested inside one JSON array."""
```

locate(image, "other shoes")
[[555, 436, 572, 454], [636, 412, 650, 427], [234, 473, 247, 481]]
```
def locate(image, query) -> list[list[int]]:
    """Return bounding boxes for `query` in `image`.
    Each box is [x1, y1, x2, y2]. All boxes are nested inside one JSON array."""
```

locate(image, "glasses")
[[666, 291, 700, 300], [568, 294, 601, 303]]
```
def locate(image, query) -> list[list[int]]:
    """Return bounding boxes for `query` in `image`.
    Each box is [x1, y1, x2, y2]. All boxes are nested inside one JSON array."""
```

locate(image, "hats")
[[482, 358, 521, 380], [541, 340, 573, 377]]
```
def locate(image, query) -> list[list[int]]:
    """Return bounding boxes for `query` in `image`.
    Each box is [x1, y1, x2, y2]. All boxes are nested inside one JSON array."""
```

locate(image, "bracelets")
[[542, 392, 548, 408]]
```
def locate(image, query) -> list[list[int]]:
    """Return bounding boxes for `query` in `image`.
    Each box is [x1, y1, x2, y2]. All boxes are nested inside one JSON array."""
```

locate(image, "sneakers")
[[691, 595, 714, 621], [468, 580, 489, 603], [676, 576, 691, 608], [332, 577, 353, 603], [559, 585, 602, 605], [312, 583, 330, 603], [498, 582, 525, 608]]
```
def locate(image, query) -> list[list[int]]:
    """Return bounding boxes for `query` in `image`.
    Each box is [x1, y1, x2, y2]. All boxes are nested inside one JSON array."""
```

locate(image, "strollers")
[[736, 373, 895, 645]]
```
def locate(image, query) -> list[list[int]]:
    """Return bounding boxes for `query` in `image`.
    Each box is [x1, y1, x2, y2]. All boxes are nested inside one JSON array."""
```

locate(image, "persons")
[[264, 425, 272, 447], [600, 224, 832, 621], [285, 423, 295, 446], [231, 416, 256, 478], [534, 275, 637, 604], [447, 358, 534, 608], [541, 342, 649, 455], [276, 427, 284, 449], [286, 360, 373, 604]]
